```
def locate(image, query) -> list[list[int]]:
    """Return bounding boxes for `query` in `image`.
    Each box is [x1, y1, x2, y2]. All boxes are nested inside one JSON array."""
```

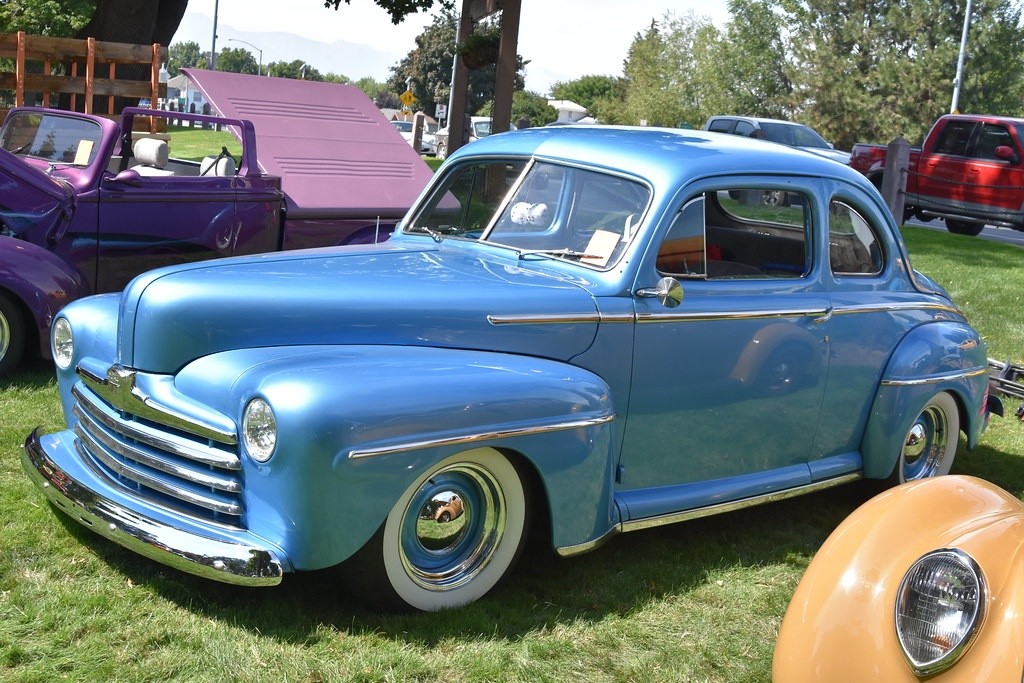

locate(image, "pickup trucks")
[[699, 117, 853, 207], [0, 68, 463, 389], [846, 115, 1024, 236]]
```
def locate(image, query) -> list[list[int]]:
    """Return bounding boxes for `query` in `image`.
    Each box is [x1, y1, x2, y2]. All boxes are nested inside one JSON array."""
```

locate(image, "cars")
[[387, 121, 434, 157], [432, 116, 605, 174], [21, 127, 1024, 617]]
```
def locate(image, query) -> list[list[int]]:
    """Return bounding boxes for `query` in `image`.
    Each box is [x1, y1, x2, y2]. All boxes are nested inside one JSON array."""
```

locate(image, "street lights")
[[228, 38, 263, 75]]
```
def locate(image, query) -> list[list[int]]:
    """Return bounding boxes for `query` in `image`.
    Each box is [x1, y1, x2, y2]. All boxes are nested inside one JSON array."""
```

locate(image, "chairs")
[[129, 137, 176, 175], [199, 152, 235, 177]]
